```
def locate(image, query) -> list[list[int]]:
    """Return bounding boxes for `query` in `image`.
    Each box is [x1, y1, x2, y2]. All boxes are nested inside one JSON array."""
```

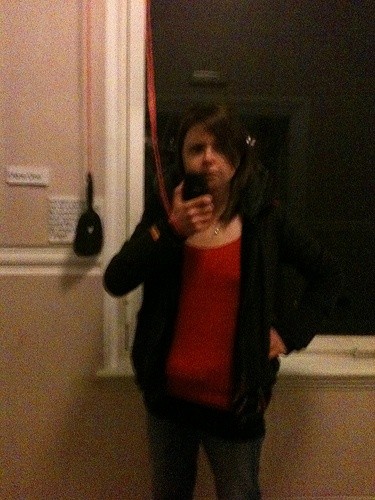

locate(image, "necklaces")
[[208, 224, 223, 235]]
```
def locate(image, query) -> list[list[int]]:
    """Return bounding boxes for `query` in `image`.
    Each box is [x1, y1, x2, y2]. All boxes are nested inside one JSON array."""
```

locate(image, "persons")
[[104, 104, 336, 500]]
